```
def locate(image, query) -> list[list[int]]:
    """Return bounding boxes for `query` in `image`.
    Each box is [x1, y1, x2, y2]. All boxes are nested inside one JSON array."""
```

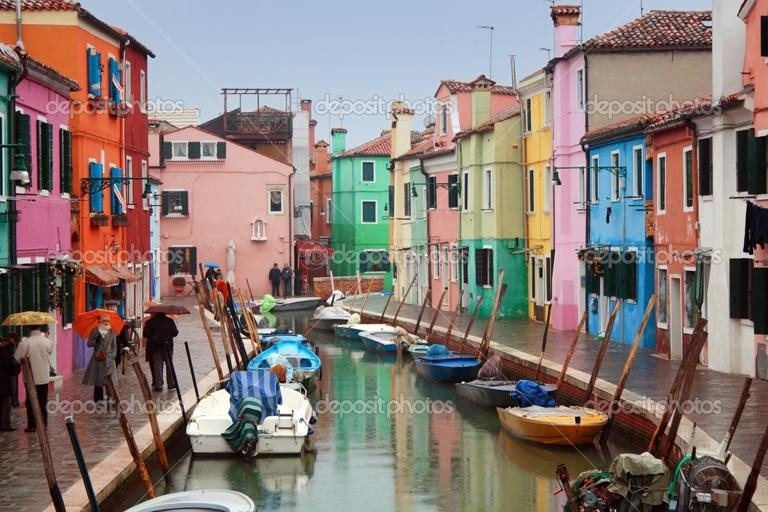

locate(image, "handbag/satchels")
[[94, 349, 106, 361]]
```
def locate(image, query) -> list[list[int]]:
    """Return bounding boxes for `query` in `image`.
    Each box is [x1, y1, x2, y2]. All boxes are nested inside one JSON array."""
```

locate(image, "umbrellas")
[[202, 263, 220, 268], [69, 307, 127, 337], [142, 305, 193, 318], [4, 310, 57, 332]]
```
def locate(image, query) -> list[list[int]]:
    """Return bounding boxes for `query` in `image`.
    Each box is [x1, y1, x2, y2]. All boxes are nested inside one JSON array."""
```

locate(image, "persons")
[[141, 310, 180, 392], [268, 262, 282, 297], [11, 327, 51, 433], [76, 315, 119, 414], [280, 262, 292, 294], [145, 339, 156, 387], [215, 268, 223, 279], [203, 267, 217, 285], [1, 332, 26, 432]]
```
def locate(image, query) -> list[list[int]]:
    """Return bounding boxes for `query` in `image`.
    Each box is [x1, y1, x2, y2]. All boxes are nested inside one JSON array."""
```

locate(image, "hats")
[[99, 314, 110, 325]]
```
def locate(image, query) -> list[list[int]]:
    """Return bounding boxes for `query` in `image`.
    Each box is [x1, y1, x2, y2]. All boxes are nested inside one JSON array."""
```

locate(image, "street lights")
[[541, 47, 550, 60], [478, 25, 494, 77]]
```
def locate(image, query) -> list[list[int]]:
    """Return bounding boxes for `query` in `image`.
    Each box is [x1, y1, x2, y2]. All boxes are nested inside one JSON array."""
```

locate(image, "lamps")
[[142, 193, 182, 211], [81, 176, 152, 199], [0, 144, 28, 180], [411, 182, 461, 198], [551, 166, 625, 185], [295, 205, 325, 217], [384, 202, 394, 210]]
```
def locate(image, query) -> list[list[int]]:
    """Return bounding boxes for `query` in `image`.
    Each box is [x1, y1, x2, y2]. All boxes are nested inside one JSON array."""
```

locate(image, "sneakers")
[[151, 385, 178, 392]]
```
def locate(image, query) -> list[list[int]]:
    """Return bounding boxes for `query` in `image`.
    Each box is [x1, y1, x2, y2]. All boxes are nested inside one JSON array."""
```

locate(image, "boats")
[[248, 340, 321, 380], [124, 489, 257, 512], [185, 382, 316, 453], [253, 296, 428, 353], [495, 407, 609, 445], [454, 380, 562, 405], [408, 344, 482, 382]]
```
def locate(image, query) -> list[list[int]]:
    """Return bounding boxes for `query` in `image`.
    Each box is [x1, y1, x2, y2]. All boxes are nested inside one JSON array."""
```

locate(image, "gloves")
[[96, 336, 101, 344]]
